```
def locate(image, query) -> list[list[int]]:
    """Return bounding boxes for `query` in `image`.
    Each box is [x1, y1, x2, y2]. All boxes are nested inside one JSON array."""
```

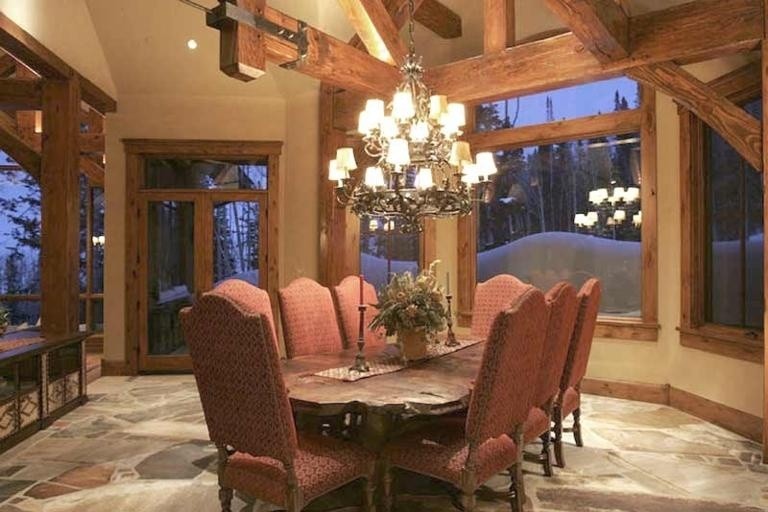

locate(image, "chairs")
[[179, 291, 378, 511], [278, 276, 344, 360], [376, 285, 550, 512], [469, 270, 535, 336], [200, 278, 279, 362], [554, 277, 602, 470], [333, 274, 387, 346], [400, 281, 578, 511]]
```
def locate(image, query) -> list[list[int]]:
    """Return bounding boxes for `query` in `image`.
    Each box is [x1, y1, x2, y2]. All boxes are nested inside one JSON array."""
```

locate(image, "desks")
[[279, 332, 487, 419]]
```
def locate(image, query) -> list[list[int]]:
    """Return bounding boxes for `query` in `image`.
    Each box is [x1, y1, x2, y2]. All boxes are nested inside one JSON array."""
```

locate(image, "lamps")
[[574, 178, 642, 232], [327, 0, 498, 235]]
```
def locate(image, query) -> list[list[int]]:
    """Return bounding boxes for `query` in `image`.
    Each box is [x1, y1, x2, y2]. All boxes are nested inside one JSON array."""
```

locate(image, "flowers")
[[366, 258, 454, 340]]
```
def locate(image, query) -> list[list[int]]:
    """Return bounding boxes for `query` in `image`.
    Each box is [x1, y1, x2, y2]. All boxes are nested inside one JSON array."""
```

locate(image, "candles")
[[443, 273, 457, 299], [354, 272, 370, 309]]
